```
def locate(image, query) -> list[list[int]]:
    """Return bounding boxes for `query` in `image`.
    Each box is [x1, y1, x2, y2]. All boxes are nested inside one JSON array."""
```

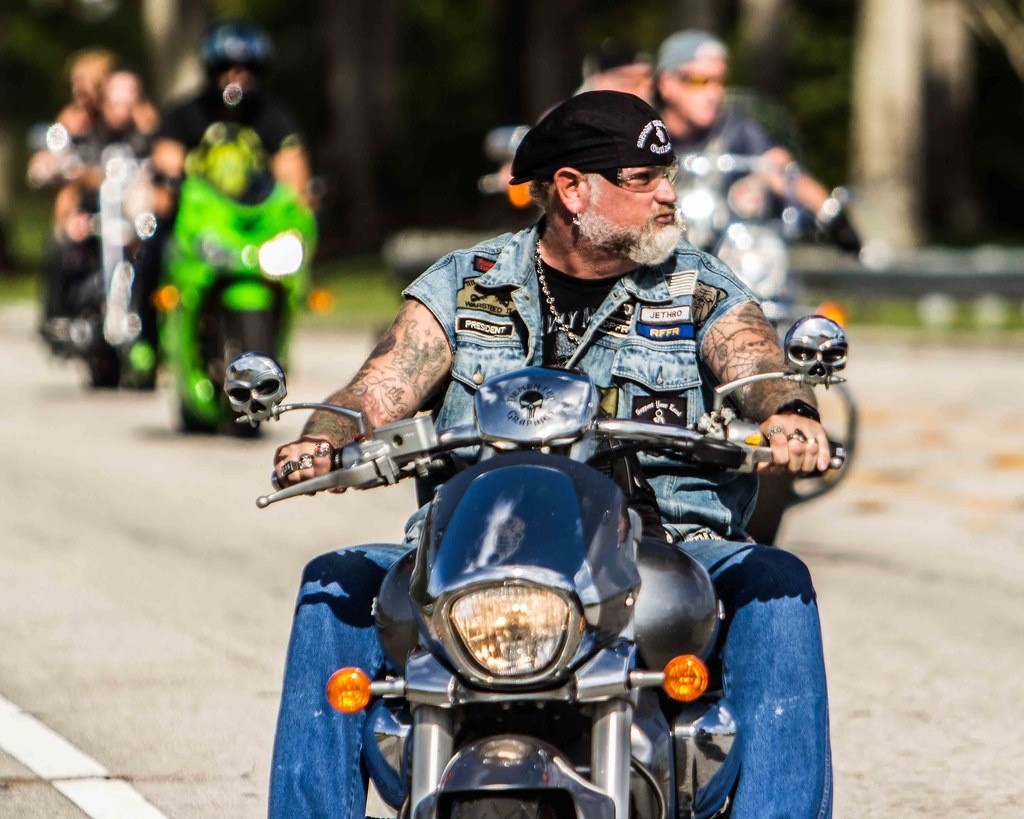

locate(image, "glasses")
[[594, 157, 680, 193], [671, 68, 727, 87]]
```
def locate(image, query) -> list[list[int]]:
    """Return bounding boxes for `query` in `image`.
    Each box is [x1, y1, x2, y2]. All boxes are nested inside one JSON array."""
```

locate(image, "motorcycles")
[[474, 131, 863, 341], [221, 314, 848, 818], [33, 118, 319, 433]]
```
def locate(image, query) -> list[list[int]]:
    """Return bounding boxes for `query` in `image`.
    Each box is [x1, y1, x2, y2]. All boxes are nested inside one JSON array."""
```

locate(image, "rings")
[[280, 461, 298, 477], [787, 434, 804, 443], [805, 438, 818, 444], [766, 427, 783, 439], [298, 454, 314, 469], [315, 442, 330, 456]]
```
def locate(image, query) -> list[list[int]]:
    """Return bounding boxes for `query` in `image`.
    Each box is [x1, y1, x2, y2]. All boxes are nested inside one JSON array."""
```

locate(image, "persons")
[[574, 31, 831, 214], [267, 89, 836, 819], [143, 27, 317, 212], [28, 51, 160, 318]]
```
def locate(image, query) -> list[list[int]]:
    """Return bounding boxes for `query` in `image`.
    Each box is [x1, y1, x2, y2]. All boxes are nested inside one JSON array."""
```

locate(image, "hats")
[[657, 27, 727, 69], [509, 90, 674, 185]]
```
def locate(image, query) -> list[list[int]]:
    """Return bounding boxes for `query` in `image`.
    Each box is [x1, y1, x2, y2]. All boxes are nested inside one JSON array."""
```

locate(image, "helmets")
[[198, 20, 272, 62]]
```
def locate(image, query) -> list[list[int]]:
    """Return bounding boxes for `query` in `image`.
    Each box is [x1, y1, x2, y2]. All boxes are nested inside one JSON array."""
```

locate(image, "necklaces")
[[535, 237, 581, 346]]
